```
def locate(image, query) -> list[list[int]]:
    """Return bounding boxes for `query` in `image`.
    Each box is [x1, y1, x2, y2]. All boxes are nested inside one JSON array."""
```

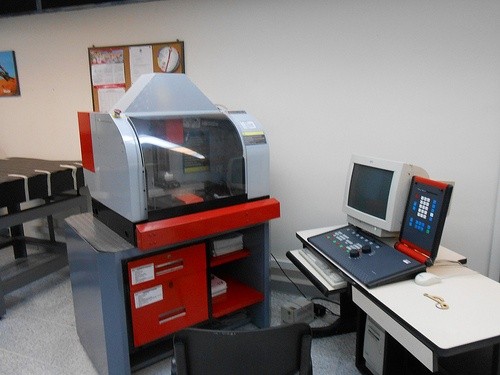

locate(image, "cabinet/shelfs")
[[64, 212, 271, 375]]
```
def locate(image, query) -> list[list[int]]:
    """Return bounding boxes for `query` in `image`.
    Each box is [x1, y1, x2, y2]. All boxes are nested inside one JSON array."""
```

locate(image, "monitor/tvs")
[[344, 155, 429, 232]]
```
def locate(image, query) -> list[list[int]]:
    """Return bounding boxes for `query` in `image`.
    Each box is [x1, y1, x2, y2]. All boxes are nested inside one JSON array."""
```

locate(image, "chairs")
[[174, 322, 313, 375]]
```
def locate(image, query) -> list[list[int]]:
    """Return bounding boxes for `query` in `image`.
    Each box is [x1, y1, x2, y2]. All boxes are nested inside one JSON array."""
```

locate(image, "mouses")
[[415, 272, 440, 286]]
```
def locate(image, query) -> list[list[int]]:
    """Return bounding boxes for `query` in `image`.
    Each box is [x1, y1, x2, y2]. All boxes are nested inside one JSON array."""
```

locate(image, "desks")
[[286, 223, 500, 375], [0, 157, 82, 321]]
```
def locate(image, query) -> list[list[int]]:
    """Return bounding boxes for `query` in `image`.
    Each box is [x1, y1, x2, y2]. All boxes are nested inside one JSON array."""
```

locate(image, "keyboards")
[[299, 248, 345, 287]]
